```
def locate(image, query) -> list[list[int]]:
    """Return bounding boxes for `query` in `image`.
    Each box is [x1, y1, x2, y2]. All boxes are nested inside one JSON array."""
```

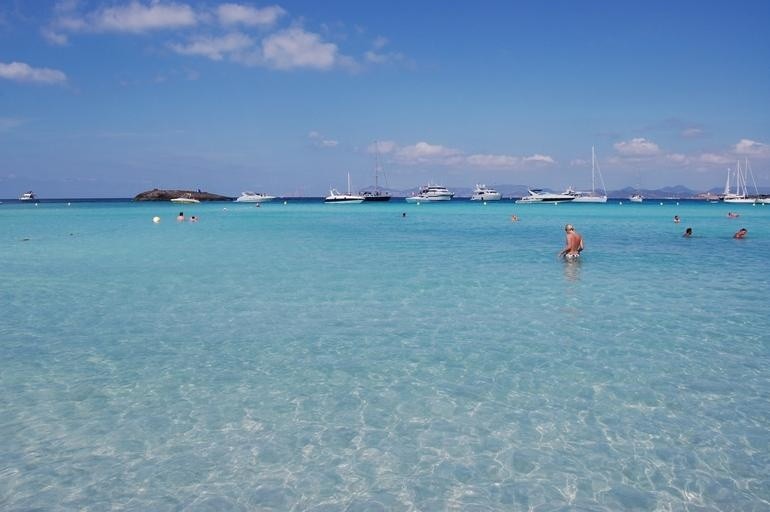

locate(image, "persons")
[[728, 212, 738, 218], [402, 212, 406, 217], [177, 211, 184, 221], [673, 215, 680, 223], [734, 228, 747, 239], [512, 215, 518, 222], [683, 227, 692, 238], [190, 216, 197, 222], [558, 224, 584, 263]]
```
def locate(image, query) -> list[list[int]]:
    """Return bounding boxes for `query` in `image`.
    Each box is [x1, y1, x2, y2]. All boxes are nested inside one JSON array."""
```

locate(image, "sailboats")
[[725, 158, 770, 204], [360, 142, 392, 201], [560, 144, 608, 202]]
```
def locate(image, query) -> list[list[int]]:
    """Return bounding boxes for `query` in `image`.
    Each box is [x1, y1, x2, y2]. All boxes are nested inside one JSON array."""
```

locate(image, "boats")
[[630, 194, 646, 202], [711, 199, 720, 203], [20, 189, 36, 202], [471, 183, 502, 201], [324, 173, 365, 203], [516, 187, 574, 204], [235, 190, 276, 203], [170, 192, 200, 205], [404, 180, 454, 202]]
